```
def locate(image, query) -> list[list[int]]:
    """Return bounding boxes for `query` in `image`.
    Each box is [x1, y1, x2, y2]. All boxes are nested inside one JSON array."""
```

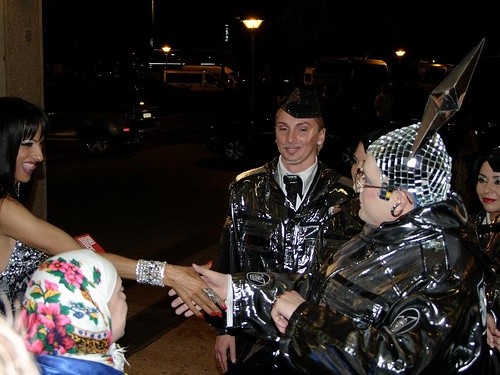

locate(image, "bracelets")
[[135, 258, 167, 288]]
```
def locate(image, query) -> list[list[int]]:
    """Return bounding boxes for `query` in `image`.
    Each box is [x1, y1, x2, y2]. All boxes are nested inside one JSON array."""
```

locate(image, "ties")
[[282, 173, 305, 210]]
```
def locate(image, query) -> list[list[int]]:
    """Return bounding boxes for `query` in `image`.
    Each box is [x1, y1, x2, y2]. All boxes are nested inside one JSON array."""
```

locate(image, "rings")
[[202, 286, 221, 305], [492, 328, 498, 336]]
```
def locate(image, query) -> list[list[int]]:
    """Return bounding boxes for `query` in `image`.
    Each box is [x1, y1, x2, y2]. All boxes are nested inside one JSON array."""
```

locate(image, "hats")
[[274, 81, 325, 120], [367, 118, 453, 207], [487, 144, 500, 173]]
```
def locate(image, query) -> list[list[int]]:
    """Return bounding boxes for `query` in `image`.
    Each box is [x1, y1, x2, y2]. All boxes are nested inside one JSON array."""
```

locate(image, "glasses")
[[355, 168, 401, 193]]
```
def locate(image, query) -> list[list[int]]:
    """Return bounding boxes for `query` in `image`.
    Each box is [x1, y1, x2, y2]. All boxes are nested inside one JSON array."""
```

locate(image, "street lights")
[[162, 45, 172, 85], [395, 48, 409, 114], [243, 18, 264, 108]]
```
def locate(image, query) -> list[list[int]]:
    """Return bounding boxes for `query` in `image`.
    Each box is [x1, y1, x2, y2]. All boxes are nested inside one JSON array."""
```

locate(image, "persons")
[[167, 84, 500, 375], [0, 95, 228, 321], [20, 248, 129, 375]]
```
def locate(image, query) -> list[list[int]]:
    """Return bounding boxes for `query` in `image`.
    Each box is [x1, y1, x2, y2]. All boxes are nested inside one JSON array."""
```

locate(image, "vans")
[[183, 66, 237, 87], [164, 70, 222, 91]]
